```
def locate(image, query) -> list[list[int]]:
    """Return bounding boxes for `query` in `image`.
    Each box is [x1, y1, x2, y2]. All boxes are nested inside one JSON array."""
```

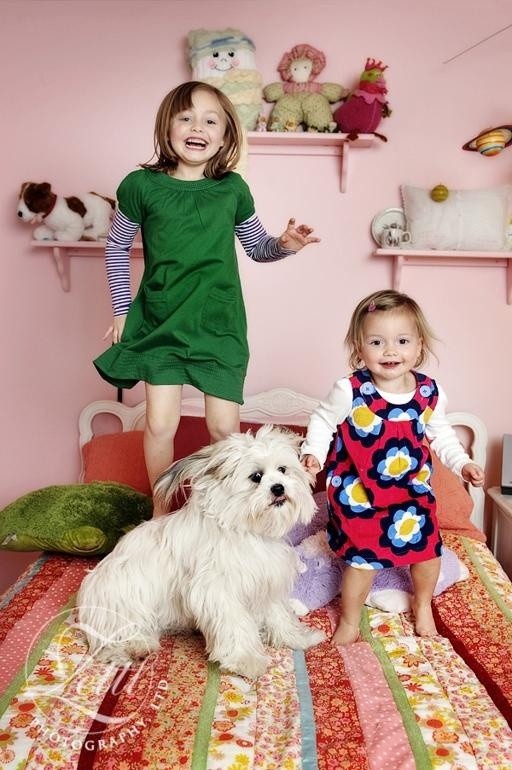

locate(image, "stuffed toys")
[[185, 27, 264, 132], [16, 181, 115, 242], [262, 43, 351, 132], [461, 123, 512, 156], [331, 57, 393, 144], [284, 489, 415, 617]]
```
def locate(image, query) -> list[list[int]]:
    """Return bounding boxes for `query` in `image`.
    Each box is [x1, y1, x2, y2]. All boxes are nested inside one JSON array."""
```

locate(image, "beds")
[[0, 386, 510, 770]]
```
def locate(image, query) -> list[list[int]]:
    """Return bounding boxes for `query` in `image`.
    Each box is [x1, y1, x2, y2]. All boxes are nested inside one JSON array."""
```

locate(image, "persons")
[[296, 288, 485, 645], [92, 80, 322, 521]]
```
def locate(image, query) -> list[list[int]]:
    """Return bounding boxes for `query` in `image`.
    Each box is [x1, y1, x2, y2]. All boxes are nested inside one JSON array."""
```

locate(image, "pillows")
[[0, 478, 155, 561], [271, 489, 476, 617]]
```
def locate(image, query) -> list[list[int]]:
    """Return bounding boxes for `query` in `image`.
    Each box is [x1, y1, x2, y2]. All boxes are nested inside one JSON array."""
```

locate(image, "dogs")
[[77, 423, 325, 680]]
